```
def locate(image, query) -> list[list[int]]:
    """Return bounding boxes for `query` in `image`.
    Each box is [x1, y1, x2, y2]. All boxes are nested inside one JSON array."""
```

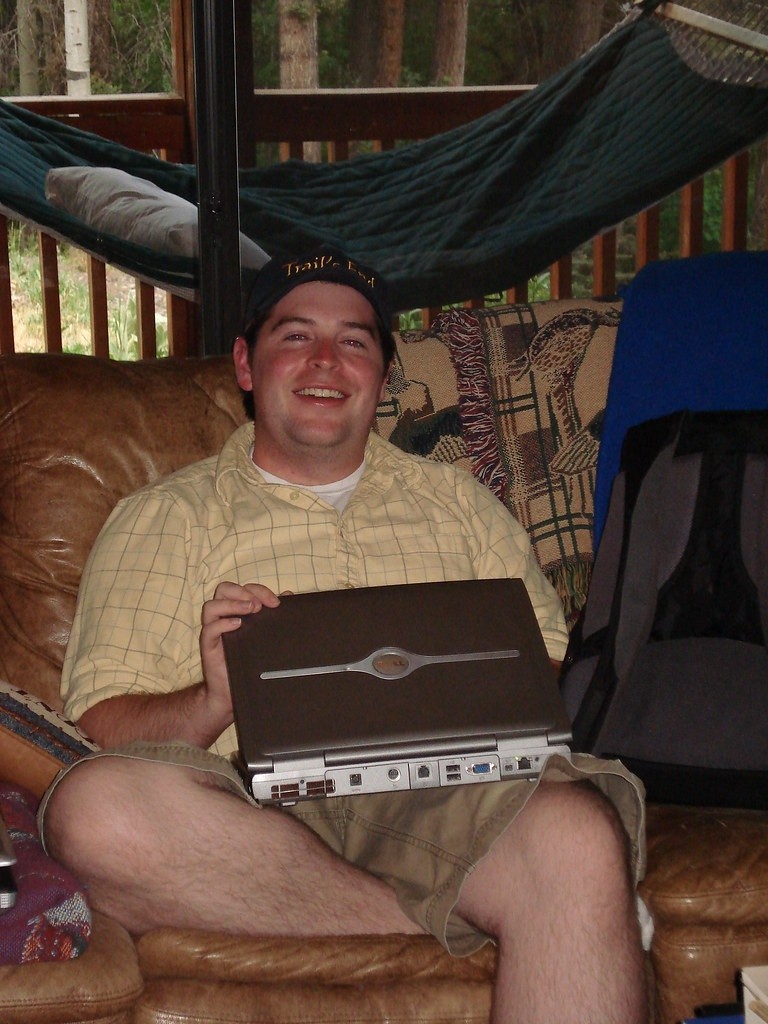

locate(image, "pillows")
[[42, 165, 272, 304]]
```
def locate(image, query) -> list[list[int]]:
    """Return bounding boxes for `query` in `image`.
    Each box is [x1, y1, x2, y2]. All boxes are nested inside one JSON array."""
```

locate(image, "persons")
[[36, 250, 647, 1024]]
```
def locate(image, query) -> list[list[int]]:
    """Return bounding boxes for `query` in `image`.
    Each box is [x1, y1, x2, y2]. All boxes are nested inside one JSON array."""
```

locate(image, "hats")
[[244, 243, 391, 353]]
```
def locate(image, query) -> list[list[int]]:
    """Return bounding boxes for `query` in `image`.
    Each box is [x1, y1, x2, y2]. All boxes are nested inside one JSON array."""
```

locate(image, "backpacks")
[[557, 410, 768, 808]]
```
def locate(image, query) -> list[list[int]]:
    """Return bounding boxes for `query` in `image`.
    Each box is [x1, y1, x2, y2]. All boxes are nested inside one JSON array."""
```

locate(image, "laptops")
[[221, 579, 573, 805]]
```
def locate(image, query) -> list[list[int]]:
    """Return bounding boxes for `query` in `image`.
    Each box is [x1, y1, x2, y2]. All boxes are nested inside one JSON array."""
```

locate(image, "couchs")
[[0, 297, 768, 1023]]
